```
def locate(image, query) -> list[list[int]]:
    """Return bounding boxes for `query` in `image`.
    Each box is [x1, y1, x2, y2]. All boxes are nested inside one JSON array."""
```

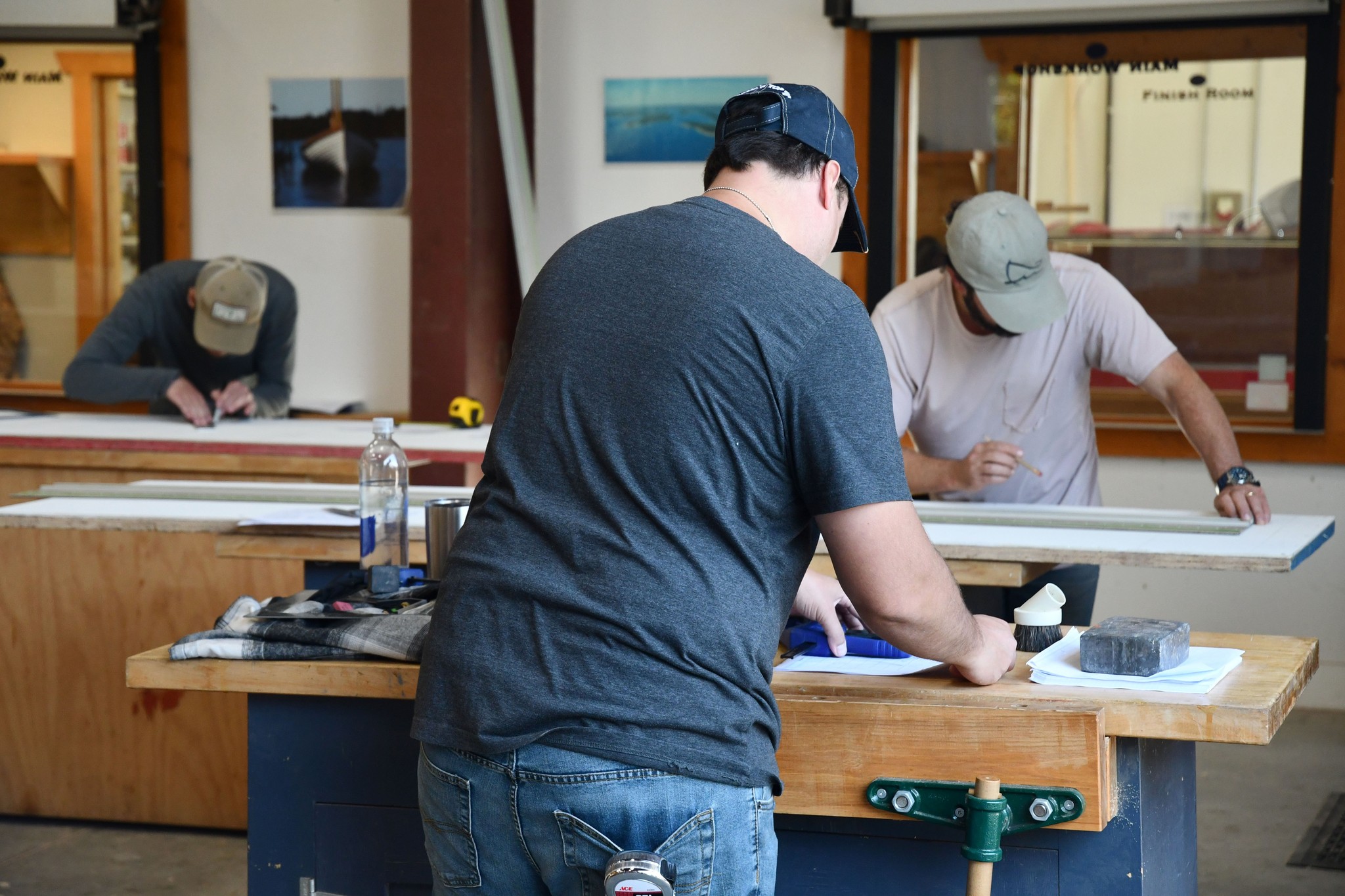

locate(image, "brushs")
[[1013, 582, 1066, 653]]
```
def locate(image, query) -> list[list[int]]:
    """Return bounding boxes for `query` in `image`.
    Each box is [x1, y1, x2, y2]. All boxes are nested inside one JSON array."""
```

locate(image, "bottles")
[[357, 417, 410, 594]]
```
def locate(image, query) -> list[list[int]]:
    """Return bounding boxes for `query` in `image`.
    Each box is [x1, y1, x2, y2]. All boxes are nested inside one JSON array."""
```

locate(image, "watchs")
[[1214, 465, 1261, 491]]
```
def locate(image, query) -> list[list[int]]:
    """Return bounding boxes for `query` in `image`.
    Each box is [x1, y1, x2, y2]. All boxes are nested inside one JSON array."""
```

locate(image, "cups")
[[424, 498, 470, 581]]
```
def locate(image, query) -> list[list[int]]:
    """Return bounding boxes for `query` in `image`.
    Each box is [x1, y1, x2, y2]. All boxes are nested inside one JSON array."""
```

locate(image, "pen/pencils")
[[984, 435, 1042, 477]]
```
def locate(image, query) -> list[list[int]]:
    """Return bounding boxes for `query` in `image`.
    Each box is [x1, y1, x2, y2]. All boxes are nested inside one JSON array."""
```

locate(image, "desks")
[[125, 629, 1321, 896]]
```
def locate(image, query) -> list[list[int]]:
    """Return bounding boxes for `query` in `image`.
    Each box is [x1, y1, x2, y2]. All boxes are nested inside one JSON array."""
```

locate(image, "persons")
[[409, 84, 1016, 895], [870, 190, 1271, 628], [61, 255, 298, 427]]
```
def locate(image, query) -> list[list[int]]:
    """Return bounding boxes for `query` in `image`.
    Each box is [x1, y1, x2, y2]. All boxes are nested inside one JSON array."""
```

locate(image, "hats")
[[192, 258, 269, 353], [716, 82, 868, 252], [947, 191, 1068, 332]]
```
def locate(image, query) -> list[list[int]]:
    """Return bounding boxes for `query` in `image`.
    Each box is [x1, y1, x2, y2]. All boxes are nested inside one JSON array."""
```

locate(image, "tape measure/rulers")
[[448, 395, 486, 429]]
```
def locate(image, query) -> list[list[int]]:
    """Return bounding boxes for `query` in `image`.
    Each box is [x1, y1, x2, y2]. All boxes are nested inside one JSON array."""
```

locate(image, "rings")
[[1246, 491, 1257, 498]]
